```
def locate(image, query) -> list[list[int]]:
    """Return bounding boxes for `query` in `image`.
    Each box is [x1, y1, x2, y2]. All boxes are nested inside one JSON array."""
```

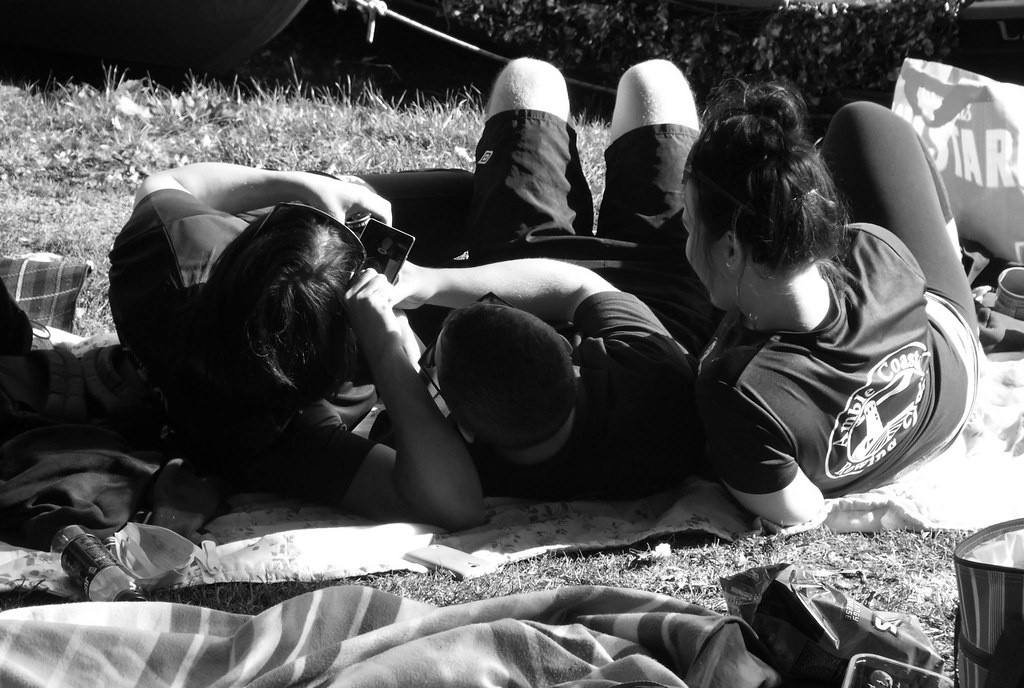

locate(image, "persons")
[[109, 163, 493, 530], [338, 55, 711, 497], [679, 83, 986, 525]]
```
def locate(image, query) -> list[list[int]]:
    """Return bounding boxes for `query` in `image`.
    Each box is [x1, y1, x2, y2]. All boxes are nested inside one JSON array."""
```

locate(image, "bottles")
[[50, 524, 147, 601]]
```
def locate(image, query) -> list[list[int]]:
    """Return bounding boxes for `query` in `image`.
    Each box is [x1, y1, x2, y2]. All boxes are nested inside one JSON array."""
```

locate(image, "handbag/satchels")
[[0, 277, 51, 356], [953, 518, 1024, 688]]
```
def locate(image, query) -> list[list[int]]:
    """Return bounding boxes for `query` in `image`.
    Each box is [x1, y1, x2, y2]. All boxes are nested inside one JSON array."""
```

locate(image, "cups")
[[993, 266, 1024, 321]]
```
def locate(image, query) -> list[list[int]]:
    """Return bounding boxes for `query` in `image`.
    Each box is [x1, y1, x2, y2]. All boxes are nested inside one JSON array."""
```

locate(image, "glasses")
[[250, 202, 367, 293], [418, 292, 518, 404], [681, 139, 751, 216]]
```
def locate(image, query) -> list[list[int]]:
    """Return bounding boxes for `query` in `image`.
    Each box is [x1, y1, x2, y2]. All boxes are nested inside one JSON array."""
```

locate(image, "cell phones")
[[353, 218, 415, 285]]
